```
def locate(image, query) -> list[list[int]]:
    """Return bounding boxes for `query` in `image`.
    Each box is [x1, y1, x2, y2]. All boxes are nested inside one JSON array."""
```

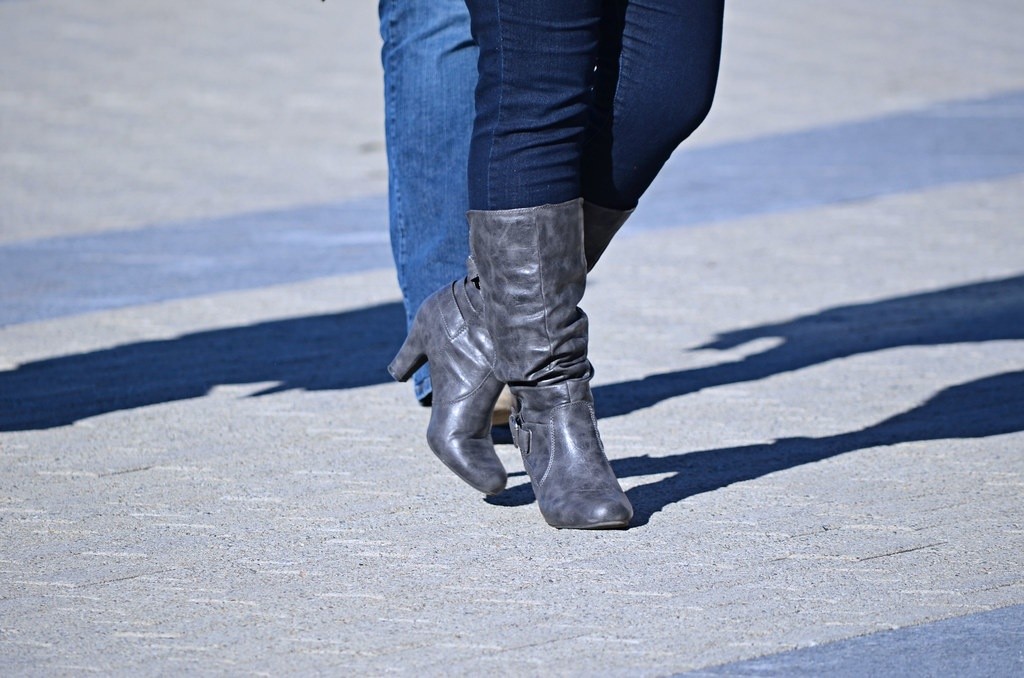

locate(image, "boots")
[[388, 197, 640, 494], [464, 197, 635, 530]]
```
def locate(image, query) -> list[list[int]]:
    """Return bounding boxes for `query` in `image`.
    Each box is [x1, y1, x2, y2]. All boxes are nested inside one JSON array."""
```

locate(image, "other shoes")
[[488, 380, 517, 430]]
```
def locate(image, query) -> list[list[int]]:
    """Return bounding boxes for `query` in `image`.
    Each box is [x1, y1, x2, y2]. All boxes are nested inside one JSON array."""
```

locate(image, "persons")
[[378, 0, 726, 531]]
